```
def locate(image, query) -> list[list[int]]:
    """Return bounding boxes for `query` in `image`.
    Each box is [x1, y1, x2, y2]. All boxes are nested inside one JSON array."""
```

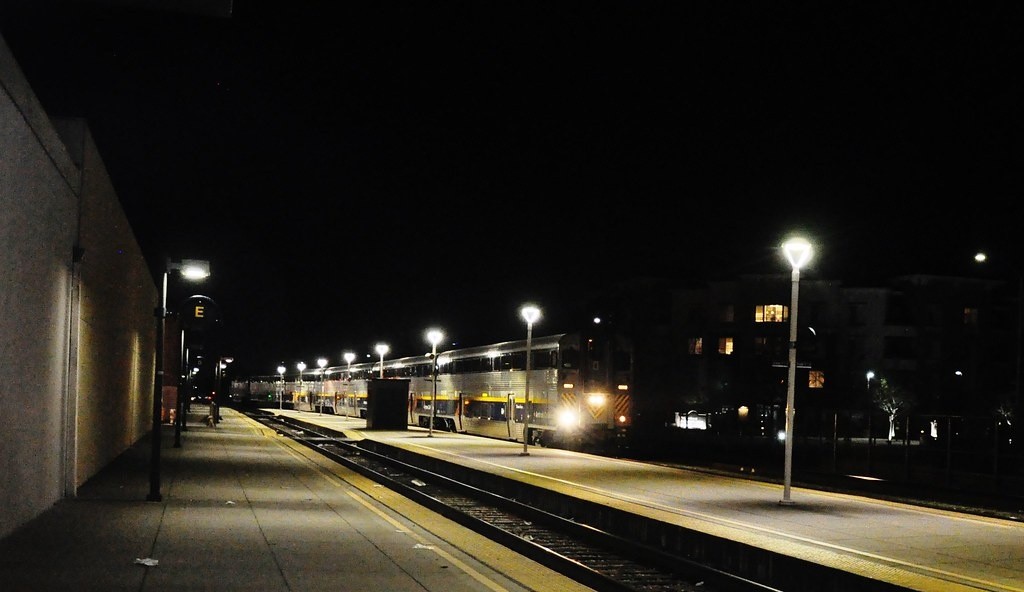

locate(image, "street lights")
[[344, 353, 357, 420], [521, 306, 541, 455], [426, 332, 443, 437], [780, 237, 815, 506], [297, 363, 307, 413], [278, 366, 285, 410], [864, 369, 876, 474], [376, 344, 389, 378], [318, 359, 328, 417], [144, 257, 213, 504]]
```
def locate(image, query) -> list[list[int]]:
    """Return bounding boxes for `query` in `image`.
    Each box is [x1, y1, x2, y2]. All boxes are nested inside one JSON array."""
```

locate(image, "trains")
[[234, 330, 635, 452]]
